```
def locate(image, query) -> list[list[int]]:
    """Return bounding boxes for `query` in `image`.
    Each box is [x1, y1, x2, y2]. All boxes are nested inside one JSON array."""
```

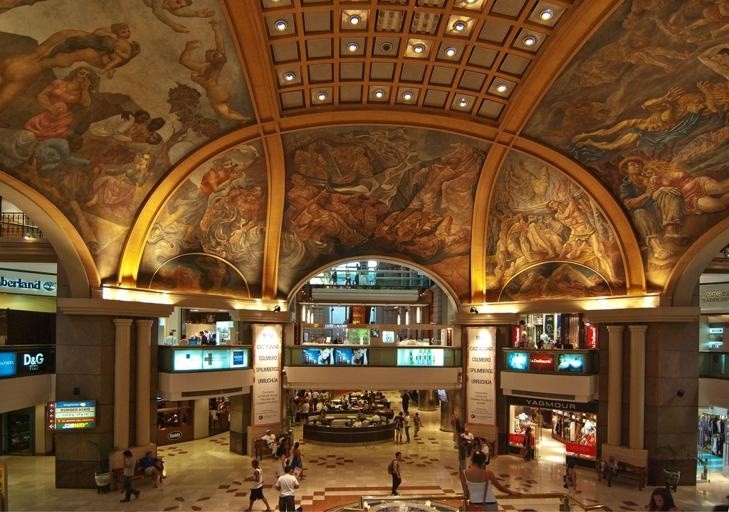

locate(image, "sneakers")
[[134, 490, 141, 500], [120, 498, 131, 503]]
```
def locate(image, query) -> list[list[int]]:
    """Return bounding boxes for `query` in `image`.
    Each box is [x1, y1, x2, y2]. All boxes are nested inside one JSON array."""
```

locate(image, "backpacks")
[[395, 416, 403, 431], [387, 459, 398, 474]]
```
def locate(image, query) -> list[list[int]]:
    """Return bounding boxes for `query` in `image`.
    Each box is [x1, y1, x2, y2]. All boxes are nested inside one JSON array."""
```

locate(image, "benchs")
[[595, 458, 648, 492], [112, 460, 165, 494], [254, 437, 291, 462], [471, 437, 497, 456]]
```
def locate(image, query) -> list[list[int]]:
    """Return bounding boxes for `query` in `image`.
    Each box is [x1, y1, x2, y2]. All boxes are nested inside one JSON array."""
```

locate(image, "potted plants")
[[87, 439, 119, 487], [662, 445, 681, 486]]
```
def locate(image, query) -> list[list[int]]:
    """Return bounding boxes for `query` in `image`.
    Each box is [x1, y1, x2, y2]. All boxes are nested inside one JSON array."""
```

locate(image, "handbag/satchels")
[[467, 502, 488, 512]]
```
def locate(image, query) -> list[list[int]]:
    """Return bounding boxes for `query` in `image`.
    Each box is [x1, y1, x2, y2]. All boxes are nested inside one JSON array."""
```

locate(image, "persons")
[[118, 448, 140, 503], [327, 263, 374, 291], [647, 485, 676, 512], [139, 450, 164, 488]]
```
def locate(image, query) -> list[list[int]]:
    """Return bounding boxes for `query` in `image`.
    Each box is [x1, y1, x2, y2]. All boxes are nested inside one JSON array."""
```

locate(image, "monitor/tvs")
[[48, 401, 96, 431]]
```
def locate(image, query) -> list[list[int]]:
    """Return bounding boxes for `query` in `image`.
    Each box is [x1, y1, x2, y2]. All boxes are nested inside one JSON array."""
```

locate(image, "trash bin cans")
[[663, 468, 680, 492], [94, 472, 111, 494]]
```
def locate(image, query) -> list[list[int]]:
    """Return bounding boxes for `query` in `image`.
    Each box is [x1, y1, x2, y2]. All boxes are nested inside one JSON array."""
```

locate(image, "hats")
[[266, 429, 271, 433]]
